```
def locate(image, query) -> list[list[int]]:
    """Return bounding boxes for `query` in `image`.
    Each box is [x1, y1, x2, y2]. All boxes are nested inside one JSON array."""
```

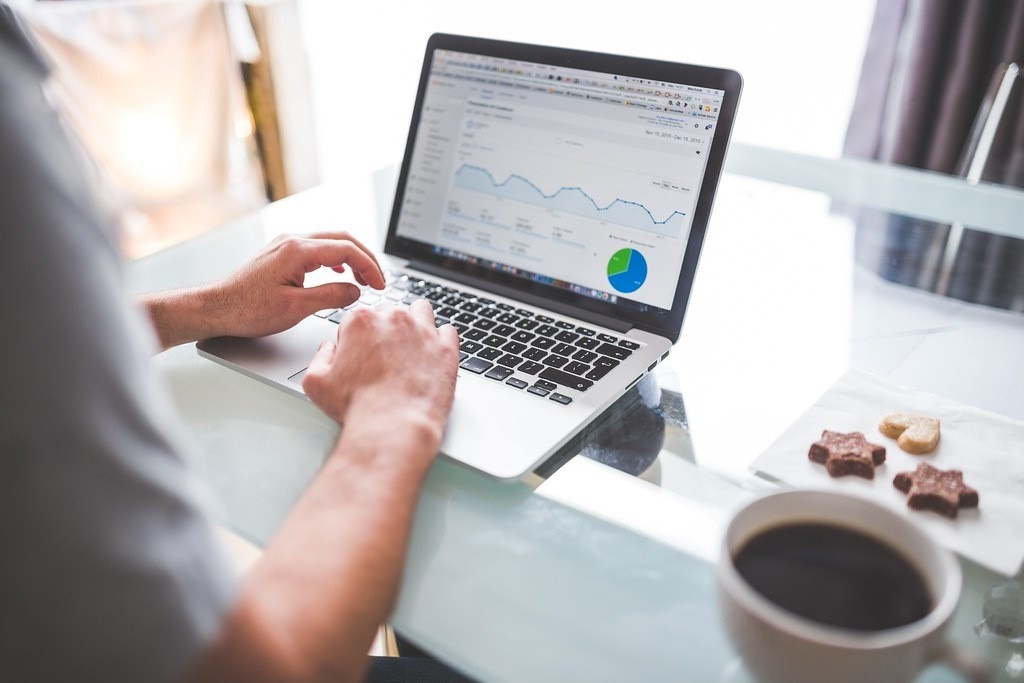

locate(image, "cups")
[[714, 487, 964, 682]]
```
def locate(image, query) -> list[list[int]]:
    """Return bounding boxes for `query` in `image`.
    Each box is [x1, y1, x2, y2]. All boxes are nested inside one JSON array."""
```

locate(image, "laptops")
[[195, 37, 743, 481]]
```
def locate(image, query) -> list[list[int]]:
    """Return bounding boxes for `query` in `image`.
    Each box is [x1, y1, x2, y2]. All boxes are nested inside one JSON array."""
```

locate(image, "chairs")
[[35, 0, 290, 266], [916, 59, 1020, 296]]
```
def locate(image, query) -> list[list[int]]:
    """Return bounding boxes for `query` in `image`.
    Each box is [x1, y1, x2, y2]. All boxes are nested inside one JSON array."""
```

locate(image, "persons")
[[0, 0, 459, 683]]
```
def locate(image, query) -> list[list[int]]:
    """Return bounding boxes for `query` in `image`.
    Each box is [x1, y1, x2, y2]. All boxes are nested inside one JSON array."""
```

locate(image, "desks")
[[130, 142, 1023, 683]]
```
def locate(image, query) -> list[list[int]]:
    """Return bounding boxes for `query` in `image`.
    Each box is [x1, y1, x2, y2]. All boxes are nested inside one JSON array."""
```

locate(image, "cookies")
[[808, 430, 886, 480], [876, 413, 940, 454], [893, 462, 979, 519]]
